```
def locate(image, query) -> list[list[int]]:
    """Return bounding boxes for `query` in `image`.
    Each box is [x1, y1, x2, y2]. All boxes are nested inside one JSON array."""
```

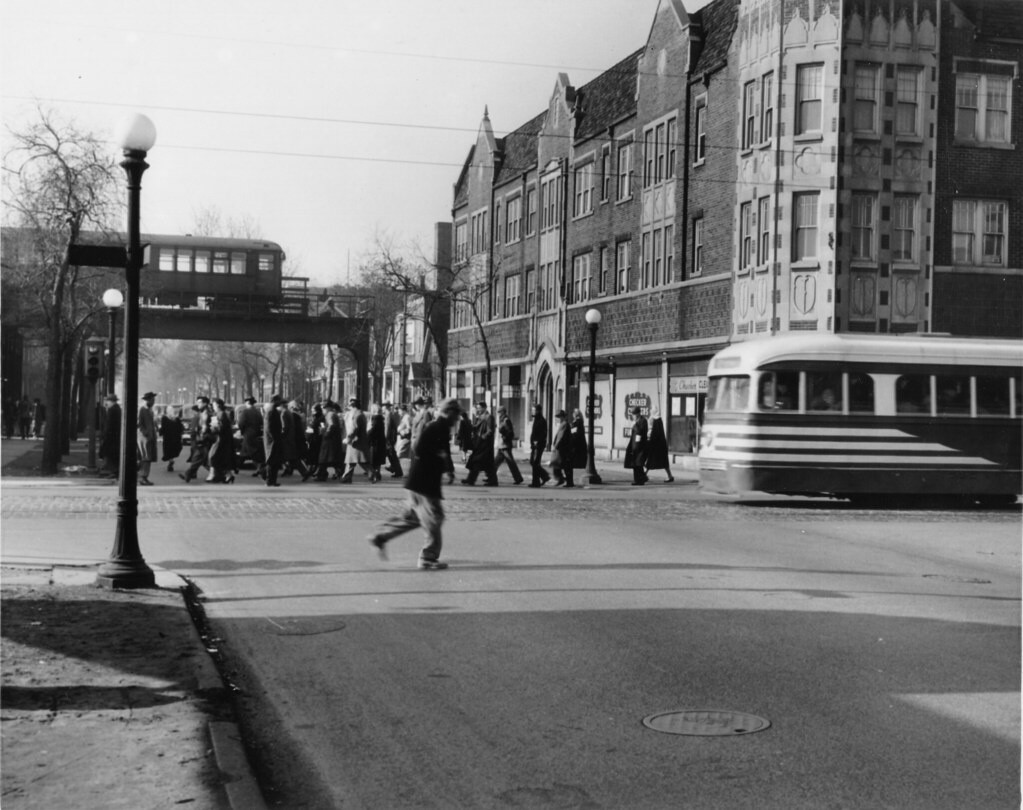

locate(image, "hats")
[[555, 410, 567, 417], [439, 397, 463, 415], [141, 393, 157, 399], [630, 406, 641, 413], [103, 394, 118, 401]]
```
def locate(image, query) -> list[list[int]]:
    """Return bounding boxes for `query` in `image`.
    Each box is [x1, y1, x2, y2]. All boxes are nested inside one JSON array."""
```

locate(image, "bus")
[[698, 333, 1023, 505]]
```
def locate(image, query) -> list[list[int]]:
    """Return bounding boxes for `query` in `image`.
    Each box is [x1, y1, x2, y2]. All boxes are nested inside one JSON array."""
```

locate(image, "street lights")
[[102, 288, 123, 394], [222, 380, 228, 402], [582, 309, 602, 485], [96, 115, 155, 591], [178, 387, 187, 404], [260, 375, 265, 403], [158, 390, 170, 404], [104, 349, 110, 397]]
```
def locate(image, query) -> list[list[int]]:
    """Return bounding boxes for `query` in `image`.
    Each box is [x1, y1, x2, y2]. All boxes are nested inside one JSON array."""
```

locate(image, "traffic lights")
[[85, 336, 105, 378]]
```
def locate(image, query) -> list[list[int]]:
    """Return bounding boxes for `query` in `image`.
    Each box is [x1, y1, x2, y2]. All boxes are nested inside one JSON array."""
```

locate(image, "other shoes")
[[144, 463, 575, 487], [632, 481, 644, 485], [367, 535, 387, 560], [418, 558, 448, 570], [664, 477, 674, 482]]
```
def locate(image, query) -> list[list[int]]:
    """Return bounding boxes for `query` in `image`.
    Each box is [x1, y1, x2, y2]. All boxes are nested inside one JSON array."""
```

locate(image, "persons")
[[644, 405, 675, 483], [527, 403, 551, 489], [810, 370, 842, 414], [137, 390, 158, 487], [461, 402, 500, 486], [95, 394, 122, 480], [548, 410, 574, 488], [570, 408, 588, 487], [1, 394, 34, 441], [178, 396, 456, 488], [482, 405, 525, 485], [366, 398, 461, 570], [157, 404, 184, 473], [774, 377, 797, 410], [455, 409, 472, 464], [624, 407, 650, 486], [34, 397, 46, 438]]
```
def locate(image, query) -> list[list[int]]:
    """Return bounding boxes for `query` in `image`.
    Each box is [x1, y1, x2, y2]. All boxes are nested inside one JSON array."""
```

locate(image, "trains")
[[1, 228, 286, 310]]
